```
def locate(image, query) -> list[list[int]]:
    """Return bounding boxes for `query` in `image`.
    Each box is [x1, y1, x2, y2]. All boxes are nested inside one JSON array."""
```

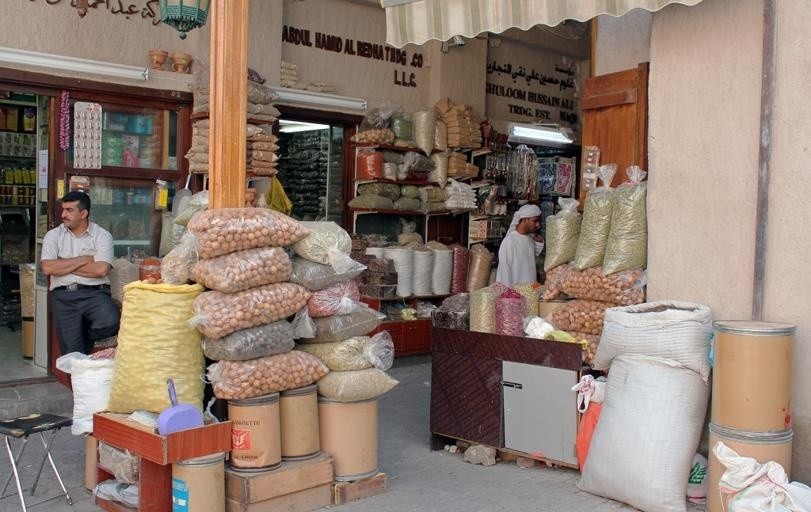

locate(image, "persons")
[[39, 191, 121, 393], [496, 205, 545, 288]]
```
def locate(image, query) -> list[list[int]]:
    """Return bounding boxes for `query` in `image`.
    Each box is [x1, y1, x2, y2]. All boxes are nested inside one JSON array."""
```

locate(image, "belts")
[[55, 284, 97, 291]]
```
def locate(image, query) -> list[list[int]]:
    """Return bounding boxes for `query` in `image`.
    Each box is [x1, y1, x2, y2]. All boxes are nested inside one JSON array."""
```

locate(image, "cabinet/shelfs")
[[90, 408, 235, 512], [361, 293, 453, 359], [352, 138, 475, 222], [1, 96, 38, 212], [63, 122, 155, 249], [427, 320, 606, 469]]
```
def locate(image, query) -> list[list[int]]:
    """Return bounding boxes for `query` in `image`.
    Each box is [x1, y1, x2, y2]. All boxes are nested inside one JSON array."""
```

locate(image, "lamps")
[[507, 121, 576, 146], [265, 84, 368, 113]]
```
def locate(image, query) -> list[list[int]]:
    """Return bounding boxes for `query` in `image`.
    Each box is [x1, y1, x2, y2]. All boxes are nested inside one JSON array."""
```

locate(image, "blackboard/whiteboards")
[[276, 129, 344, 217]]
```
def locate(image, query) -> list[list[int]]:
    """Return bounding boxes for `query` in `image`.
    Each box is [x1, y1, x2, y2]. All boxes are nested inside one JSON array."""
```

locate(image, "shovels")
[[171, 173, 192, 216], [157, 379, 202, 436]]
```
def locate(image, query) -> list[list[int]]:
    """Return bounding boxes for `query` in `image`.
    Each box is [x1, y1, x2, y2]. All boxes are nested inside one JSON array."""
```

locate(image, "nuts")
[[543, 264, 645, 365], [186, 207, 328, 400]]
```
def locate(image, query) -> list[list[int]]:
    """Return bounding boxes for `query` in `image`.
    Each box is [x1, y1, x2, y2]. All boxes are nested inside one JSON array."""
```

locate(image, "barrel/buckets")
[[279, 385, 321, 457], [172, 451, 226, 512], [317, 396, 380, 477], [228, 392, 282, 468]]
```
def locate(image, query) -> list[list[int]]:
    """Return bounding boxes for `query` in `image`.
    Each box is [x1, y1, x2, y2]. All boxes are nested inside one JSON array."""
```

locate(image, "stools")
[[0, 412, 76, 512]]
